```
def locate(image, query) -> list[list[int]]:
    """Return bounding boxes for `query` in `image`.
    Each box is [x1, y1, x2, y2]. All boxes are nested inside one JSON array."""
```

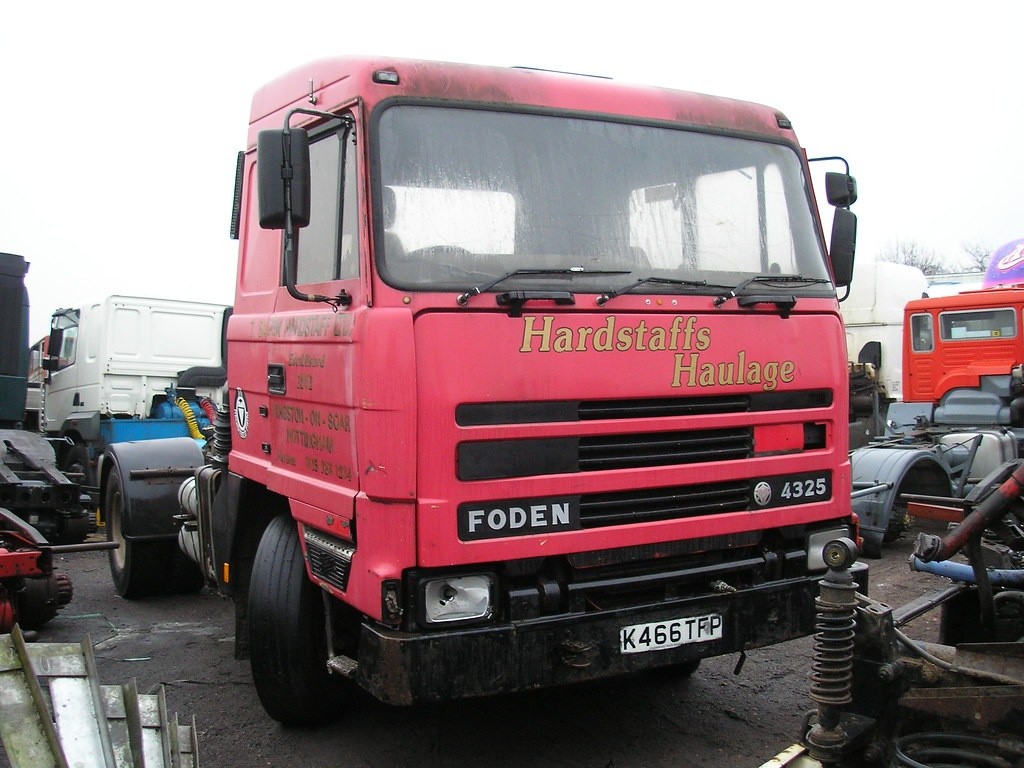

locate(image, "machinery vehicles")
[[0, 250, 97, 634]]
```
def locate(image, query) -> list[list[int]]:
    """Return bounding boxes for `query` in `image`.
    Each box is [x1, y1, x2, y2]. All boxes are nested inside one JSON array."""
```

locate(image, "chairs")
[[381, 184, 404, 262]]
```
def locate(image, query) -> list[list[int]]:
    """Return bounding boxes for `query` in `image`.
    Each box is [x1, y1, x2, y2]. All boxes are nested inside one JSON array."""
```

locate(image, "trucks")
[[93, 56, 855, 730], [819, 256, 1023, 560], [29, 291, 234, 498]]
